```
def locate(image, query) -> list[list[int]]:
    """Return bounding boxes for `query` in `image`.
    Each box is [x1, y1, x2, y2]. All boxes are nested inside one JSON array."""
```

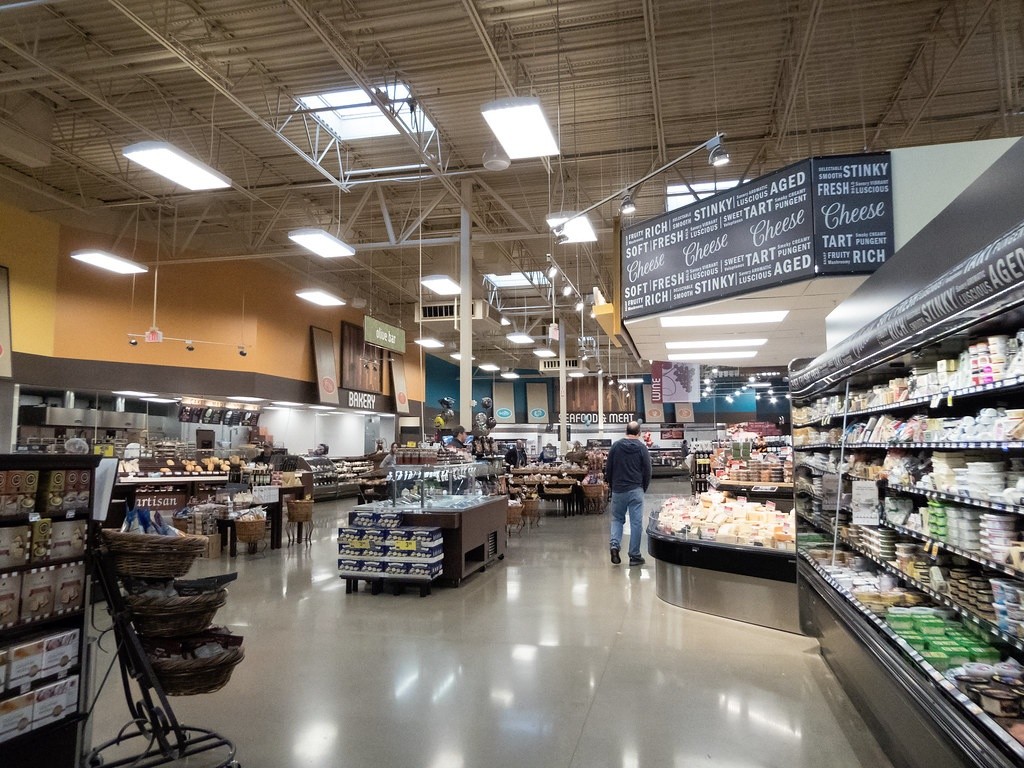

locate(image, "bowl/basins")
[[810, 335, 1024, 719]]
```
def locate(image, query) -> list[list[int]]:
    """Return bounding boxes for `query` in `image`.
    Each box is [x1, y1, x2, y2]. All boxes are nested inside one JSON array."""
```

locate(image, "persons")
[[447, 426, 466, 448], [379, 442, 401, 468], [605, 421, 651, 566], [251, 444, 277, 466], [681, 440, 688, 456], [504, 440, 528, 468], [536, 443, 557, 464]]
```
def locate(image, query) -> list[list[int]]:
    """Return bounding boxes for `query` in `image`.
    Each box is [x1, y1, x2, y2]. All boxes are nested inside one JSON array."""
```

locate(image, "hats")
[[263, 441, 273, 448]]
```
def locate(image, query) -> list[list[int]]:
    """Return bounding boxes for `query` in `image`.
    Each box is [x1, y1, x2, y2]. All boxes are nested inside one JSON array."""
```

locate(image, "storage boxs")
[[0, 468, 86, 743], [338, 525, 443, 578]]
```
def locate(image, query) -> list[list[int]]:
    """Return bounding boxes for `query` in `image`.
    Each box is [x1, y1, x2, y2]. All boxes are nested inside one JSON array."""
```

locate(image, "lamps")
[[68, 0, 731, 380]]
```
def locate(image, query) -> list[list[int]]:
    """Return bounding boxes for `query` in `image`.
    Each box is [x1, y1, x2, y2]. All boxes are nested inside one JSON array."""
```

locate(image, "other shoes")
[[629, 558, 645, 565], [611, 548, 621, 564]]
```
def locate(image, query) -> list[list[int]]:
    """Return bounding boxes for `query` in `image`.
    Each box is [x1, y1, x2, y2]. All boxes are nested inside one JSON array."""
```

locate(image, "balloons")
[[434, 397, 455, 429], [473, 397, 497, 437], [643, 431, 653, 448]]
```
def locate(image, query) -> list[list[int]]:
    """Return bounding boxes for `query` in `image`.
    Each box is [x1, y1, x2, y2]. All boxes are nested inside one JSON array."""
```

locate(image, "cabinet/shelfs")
[[0, 450, 103, 768], [306, 455, 377, 499]]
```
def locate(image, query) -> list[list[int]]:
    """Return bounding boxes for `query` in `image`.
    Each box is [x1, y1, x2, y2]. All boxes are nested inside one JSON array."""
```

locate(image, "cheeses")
[[657, 492, 796, 552]]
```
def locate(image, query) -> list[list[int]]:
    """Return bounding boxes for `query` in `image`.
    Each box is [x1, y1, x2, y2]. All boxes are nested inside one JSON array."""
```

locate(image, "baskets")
[[234, 519, 265, 543], [509, 468, 588, 495], [583, 483, 602, 499], [123, 581, 229, 640], [522, 499, 538, 517], [103, 526, 209, 579], [507, 504, 525, 524], [146, 641, 245, 696], [285, 500, 314, 522]]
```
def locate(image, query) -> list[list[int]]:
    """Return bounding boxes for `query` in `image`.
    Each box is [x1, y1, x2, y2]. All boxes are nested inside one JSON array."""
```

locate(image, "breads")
[[160, 456, 231, 472]]
